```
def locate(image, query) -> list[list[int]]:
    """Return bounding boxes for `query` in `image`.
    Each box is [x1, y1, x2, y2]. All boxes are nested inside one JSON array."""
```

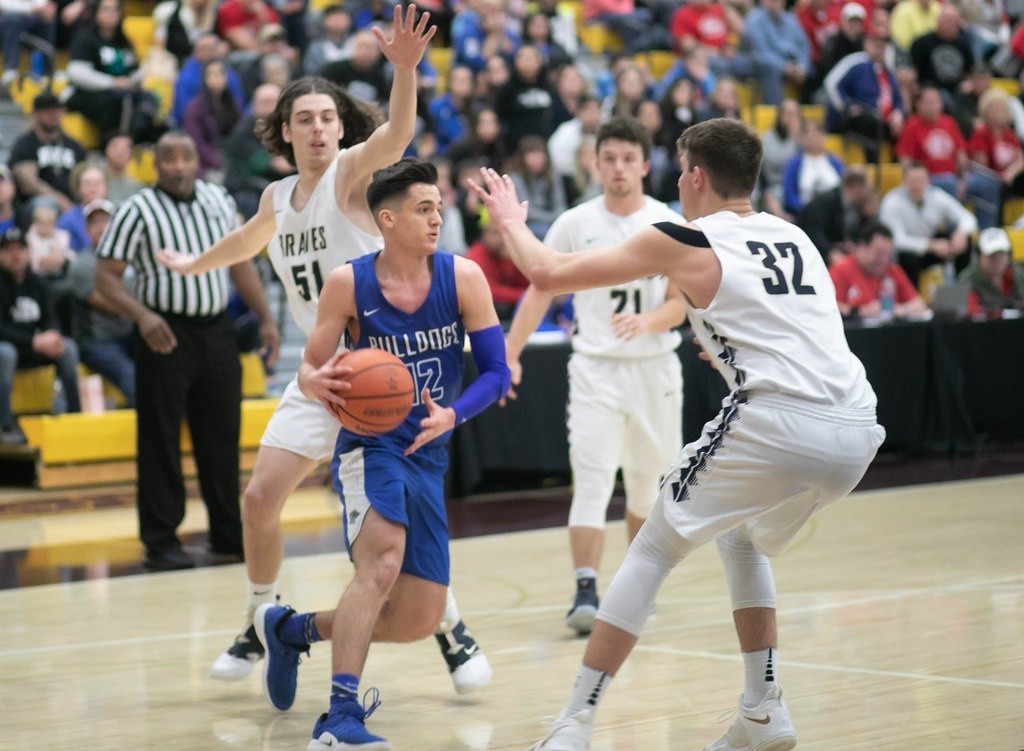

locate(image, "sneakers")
[[699, 684, 797, 751], [525, 709, 594, 751], [210, 620, 268, 681], [562, 588, 600, 638], [306, 685, 390, 751], [254, 603, 312, 713], [433, 614, 493, 693]]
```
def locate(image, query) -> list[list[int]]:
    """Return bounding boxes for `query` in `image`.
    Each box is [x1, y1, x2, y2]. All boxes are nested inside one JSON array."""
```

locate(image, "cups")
[[76, 373, 107, 413]]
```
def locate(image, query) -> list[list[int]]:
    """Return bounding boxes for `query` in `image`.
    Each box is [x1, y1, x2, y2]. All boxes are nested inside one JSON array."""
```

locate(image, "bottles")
[[878, 279, 897, 326]]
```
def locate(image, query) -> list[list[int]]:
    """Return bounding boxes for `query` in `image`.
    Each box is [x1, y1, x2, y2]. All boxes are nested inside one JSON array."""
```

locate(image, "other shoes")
[[140, 547, 194, 570], [208, 544, 245, 564]]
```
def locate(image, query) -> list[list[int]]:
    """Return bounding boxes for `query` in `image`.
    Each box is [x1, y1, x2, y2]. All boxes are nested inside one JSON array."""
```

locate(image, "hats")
[[840, 3, 860, 15], [33, 95, 64, 108], [83, 200, 116, 219], [0, 227, 27, 249], [974, 227, 1011, 252]]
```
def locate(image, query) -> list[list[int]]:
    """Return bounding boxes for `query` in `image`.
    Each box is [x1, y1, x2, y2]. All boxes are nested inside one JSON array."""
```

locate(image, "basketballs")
[[330, 348, 415, 435]]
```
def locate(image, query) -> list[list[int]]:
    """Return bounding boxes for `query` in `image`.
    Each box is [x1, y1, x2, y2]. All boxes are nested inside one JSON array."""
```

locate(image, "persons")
[[255, 159, 511, 751], [96, 132, 281, 569], [466, 117, 886, 751], [155, 7, 490, 695], [498, 119, 687, 632], [0, 0, 1024, 446]]
